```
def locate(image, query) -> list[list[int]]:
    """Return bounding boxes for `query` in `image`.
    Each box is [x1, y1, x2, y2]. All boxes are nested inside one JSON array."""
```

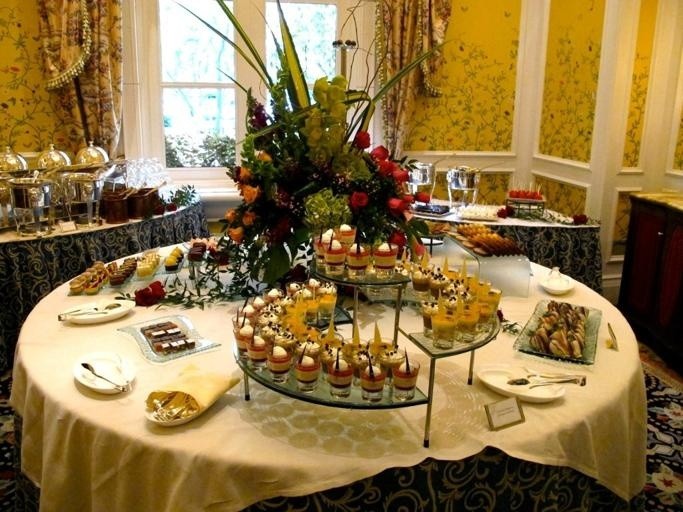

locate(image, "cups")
[[100, 190, 151, 224], [229, 222, 500, 401]]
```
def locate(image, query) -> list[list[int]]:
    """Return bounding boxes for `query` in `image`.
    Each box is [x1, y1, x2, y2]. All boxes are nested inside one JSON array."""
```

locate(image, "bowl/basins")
[[70, 346, 139, 397]]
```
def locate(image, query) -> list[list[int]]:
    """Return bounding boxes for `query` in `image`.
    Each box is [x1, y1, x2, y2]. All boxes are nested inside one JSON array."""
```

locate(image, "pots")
[[5, 175, 56, 239], [62, 170, 103, 231], [444, 165, 481, 214], [405, 161, 436, 208]]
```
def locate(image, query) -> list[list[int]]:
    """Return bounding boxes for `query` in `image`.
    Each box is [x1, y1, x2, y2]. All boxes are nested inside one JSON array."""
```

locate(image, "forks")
[[547, 265, 561, 282]]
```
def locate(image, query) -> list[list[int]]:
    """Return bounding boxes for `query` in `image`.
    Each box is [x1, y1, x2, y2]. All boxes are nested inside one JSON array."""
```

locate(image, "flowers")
[[168, 1, 462, 301]]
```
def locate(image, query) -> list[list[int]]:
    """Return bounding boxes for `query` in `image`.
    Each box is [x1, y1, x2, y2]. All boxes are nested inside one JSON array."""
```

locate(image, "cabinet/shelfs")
[[616, 187, 681, 376]]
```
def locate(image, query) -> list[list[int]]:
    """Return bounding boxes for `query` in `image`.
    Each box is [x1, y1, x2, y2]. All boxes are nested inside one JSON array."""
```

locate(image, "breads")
[[447, 223, 525, 258]]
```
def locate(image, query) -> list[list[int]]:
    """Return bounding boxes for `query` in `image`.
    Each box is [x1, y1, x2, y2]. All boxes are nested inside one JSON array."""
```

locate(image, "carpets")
[[0, 333, 680, 510]]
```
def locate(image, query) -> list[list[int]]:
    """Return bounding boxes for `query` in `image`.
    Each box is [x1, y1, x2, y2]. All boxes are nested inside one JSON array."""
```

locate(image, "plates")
[[114, 310, 225, 368], [476, 365, 568, 405], [454, 206, 498, 221], [538, 274, 578, 297], [407, 203, 451, 217], [510, 297, 601, 364], [49, 296, 137, 328], [504, 190, 551, 205]]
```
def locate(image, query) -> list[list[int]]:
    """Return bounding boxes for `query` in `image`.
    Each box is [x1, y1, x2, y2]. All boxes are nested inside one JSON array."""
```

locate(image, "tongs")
[[56, 304, 110, 323], [80, 358, 130, 393], [505, 371, 587, 392]]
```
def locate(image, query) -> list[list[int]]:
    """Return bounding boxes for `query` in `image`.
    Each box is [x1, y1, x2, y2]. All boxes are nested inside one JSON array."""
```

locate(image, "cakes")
[[69, 241, 206, 294]]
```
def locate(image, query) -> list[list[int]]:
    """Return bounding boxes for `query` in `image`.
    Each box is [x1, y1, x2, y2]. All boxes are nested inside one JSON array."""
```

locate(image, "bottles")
[[125, 155, 166, 187]]
